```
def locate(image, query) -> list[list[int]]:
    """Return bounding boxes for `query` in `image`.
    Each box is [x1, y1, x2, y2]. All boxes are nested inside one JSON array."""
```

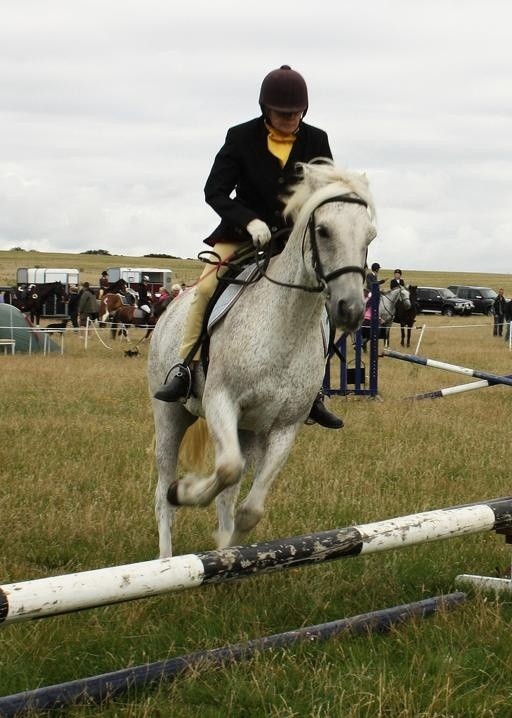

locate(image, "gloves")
[[246, 218, 272, 247]]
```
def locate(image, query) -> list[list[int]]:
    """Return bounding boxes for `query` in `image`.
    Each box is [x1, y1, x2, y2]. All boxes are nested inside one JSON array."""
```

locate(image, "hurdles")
[[379, 346, 510, 400], [0, 495, 512, 718]]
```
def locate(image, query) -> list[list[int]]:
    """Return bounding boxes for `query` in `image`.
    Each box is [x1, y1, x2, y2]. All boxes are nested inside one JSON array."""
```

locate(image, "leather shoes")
[[154, 370, 191, 402], [309, 399, 343, 429]]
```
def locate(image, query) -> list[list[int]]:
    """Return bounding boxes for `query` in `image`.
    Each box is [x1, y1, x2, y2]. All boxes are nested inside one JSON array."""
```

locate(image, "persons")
[[366, 263, 389, 294], [154, 66, 344, 429], [493, 287, 506, 336], [0, 271, 182, 339], [390, 269, 404, 290], [505, 298, 512, 341], [353, 288, 372, 352]]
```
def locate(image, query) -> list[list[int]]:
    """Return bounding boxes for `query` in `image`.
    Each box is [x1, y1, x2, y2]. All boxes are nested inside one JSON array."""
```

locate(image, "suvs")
[[447, 284, 510, 316], [416, 287, 475, 318]]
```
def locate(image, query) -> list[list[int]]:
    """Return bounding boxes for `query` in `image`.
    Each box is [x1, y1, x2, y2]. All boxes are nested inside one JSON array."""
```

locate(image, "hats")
[[102, 271, 108, 275], [394, 269, 401, 274]]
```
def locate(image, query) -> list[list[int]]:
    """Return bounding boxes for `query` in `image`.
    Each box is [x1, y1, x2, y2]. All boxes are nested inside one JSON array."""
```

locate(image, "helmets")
[[259, 65, 308, 114], [372, 263, 380, 271]]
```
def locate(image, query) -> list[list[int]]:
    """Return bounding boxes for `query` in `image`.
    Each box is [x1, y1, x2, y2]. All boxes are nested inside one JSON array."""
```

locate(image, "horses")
[[3, 279, 70, 328], [357, 283, 421, 347], [86, 278, 167, 344], [142, 154, 382, 560]]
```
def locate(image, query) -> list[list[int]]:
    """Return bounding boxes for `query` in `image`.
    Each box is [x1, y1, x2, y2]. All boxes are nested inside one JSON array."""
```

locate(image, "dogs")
[[123, 346, 142, 359], [39, 317, 71, 337]]
